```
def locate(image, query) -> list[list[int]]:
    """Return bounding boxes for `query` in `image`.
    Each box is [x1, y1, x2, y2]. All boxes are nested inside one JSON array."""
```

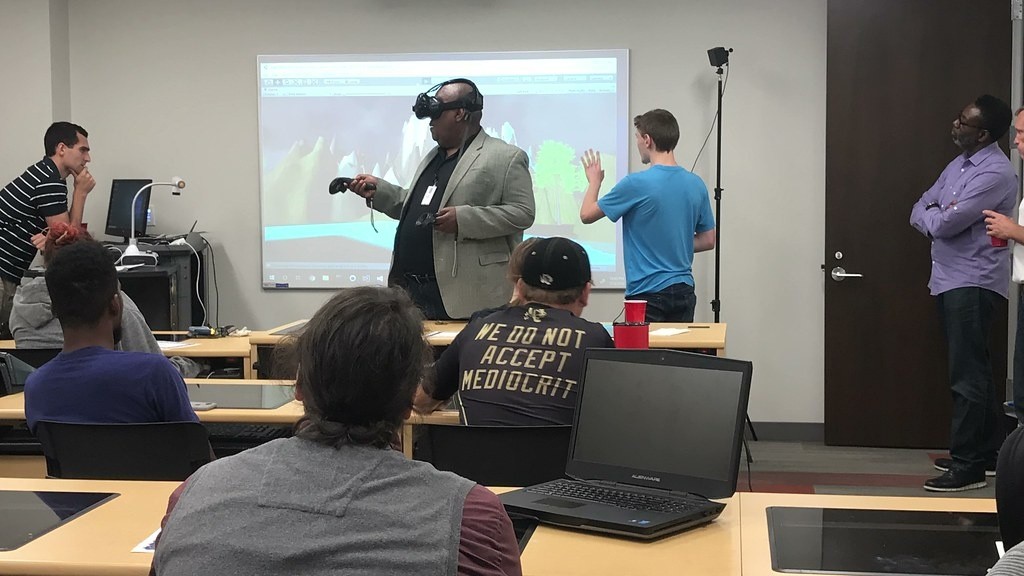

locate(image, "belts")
[[397, 266, 436, 287]]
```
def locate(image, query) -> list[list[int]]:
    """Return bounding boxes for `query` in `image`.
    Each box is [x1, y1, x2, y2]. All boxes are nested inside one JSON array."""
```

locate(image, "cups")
[[614, 322, 651, 350], [624, 300, 647, 324], [991, 234, 1007, 247]]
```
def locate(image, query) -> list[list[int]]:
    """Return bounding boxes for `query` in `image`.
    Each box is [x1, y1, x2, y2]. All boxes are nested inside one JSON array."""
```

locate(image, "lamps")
[[125, 177, 186, 258]]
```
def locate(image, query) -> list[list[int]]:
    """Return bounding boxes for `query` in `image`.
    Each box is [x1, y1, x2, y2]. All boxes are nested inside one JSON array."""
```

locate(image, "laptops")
[[492, 349, 753, 536]]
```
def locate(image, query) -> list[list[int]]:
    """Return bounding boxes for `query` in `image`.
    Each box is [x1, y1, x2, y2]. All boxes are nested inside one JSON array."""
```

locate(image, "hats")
[[521, 236, 595, 290]]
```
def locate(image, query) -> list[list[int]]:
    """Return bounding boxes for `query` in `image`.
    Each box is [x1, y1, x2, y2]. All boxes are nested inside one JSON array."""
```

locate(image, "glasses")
[[956, 110, 993, 137]]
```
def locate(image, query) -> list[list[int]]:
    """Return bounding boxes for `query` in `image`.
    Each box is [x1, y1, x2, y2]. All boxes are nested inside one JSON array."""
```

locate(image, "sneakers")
[[923, 466, 987, 491], [934, 458, 997, 476]]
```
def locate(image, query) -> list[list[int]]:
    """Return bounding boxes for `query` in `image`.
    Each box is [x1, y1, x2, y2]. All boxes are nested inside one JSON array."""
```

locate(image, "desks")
[[0, 479, 1001, 576], [0, 319, 727, 381], [0, 389, 463, 473], [23, 237, 210, 332]]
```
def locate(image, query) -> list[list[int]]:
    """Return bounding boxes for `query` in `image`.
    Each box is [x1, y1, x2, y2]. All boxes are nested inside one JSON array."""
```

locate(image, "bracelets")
[[926, 203, 939, 211]]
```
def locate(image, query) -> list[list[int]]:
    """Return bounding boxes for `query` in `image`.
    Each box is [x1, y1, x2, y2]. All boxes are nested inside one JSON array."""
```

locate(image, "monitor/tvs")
[[106, 179, 152, 244]]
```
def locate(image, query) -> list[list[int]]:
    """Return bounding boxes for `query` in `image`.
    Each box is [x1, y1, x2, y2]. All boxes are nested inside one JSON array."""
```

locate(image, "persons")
[[981, 108, 1024, 423], [9, 225, 202, 377], [580, 108, 715, 323], [0, 122, 96, 341], [344, 79, 536, 320], [149, 285, 522, 576], [415, 237, 616, 425], [910, 96, 1018, 492], [24, 240, 217, 518]]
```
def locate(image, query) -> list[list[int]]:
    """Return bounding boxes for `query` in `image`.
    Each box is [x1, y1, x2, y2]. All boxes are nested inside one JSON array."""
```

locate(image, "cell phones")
[[190, 401, 217, 411]]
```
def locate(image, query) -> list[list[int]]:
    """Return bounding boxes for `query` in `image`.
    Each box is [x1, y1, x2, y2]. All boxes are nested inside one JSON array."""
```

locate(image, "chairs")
[[414, 421, 572, 486], [35, 420, 208, 488], [0, 347, 64, 371]]
